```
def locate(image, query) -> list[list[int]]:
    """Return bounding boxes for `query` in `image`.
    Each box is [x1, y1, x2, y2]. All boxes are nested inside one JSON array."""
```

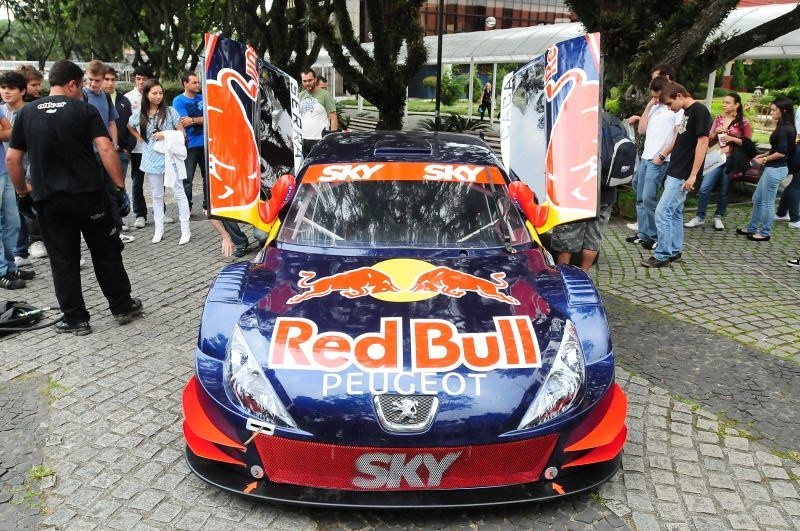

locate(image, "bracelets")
[[191, 117, 195, 126], [658, 154, 665, 161], [113, 143, 119, 150], [763, 156, 768, 164]]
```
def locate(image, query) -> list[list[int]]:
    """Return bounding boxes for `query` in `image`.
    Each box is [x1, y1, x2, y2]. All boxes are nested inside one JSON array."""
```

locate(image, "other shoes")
[[736, 227, 755, 235], [746, 232, 770, 241]]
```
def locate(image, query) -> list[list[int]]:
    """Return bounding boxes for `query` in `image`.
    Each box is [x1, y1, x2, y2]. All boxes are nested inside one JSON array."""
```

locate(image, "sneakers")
[[641, 239, 658, 249], [683, 217, 705, 228], [163, 211, 174, 223], [53, 321, 93, 336], [118, 233, 135, 243], [28, 240, 48, 258], [712, 216, 724, 230], [785, 257, 800, 268], [134, 216, 148, 229], [625, 221, 639, 230], [624, 235, 644, 243], [11, 255, 31, 269], [669, 253, 682, 261], [773, 213, 791, 221], [121, 219, 130, 231], [641, 256, 671, 268], [113, 297, 143, 325], [7, 269, 35, 279], [0, 275, 26, 291], [787, 220, 800, 228]]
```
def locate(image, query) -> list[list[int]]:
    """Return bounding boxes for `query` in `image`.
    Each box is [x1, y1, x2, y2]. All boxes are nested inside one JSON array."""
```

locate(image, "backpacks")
[[598, 115, 636, 190]]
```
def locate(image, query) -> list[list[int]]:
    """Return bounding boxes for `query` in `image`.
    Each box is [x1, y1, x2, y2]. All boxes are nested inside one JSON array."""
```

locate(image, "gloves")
[[14, 190, 38, 220], [115, 186, 132, 218]]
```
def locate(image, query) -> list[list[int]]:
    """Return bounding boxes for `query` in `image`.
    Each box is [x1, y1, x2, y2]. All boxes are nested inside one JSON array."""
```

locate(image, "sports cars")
[[182, 129, 627, 510]]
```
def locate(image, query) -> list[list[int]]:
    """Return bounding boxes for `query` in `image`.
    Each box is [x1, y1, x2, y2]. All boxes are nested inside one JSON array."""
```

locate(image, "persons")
[[170, 71, 212, 221], [314, 75, 328, 89], [204, 204, 270, 259], [478, 82, 492, 124], [126, 79, 192, 246], [297, 67, 339, 164], [772, 103, 800, 229], [684, 91, 753, 232], [547, 81, 628, 280], [77, 60, 135, 244], [0, 69, 49, 267], [627, 65, 674, 233], [625, 75, 684, 248], [639, 82, 712, 270], [786, 256, 800, 268], [121, 66, 175, 229], [734, 94, 798, 242], [4, 58, 143, 336], [101, 65, 137, 231], [12, 62, 44, 98], [0, 104, 38, 289]]
